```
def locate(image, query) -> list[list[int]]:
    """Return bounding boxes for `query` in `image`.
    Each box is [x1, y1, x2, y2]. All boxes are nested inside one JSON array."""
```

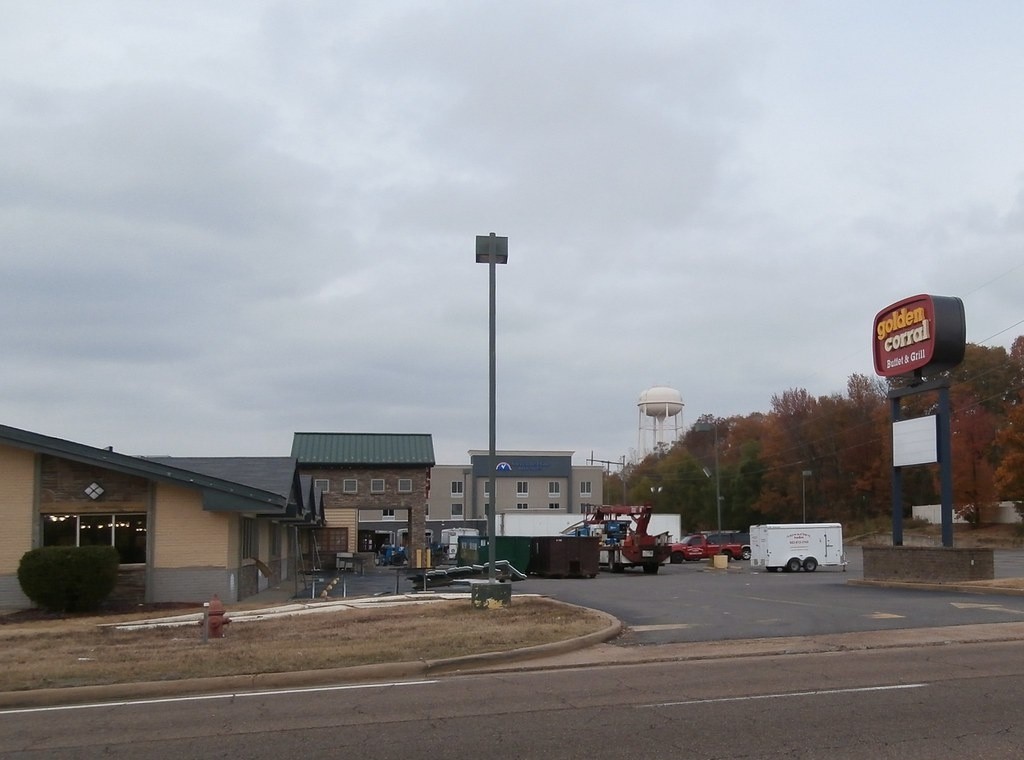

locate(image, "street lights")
[[802, 470, 813, 524], [474, 230, 509, 577]]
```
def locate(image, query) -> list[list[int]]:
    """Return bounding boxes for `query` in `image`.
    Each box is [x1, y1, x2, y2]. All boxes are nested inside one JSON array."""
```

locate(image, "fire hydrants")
[[198, 592, 234, 638]]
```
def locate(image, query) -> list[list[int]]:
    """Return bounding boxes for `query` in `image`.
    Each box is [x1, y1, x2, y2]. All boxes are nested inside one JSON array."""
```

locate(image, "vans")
[[704, 532, 752, 560]]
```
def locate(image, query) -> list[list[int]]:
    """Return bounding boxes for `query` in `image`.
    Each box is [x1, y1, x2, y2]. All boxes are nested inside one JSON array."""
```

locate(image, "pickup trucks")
[[670, 534, 743, 562]]
